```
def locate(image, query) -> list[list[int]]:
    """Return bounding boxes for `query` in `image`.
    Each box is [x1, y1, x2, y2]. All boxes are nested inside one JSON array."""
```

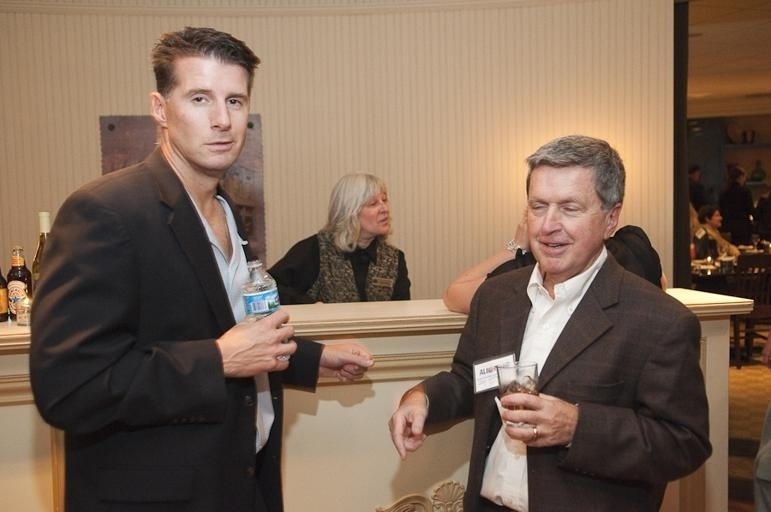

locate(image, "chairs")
[[736, 252, 771, 367]]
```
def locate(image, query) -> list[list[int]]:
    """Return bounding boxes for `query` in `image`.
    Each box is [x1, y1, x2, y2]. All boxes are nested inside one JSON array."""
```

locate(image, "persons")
[[693, 203, 741, 259], [717, 166, 754, 245], [442, 212, 669, 320], [265, 172, 413, 305], [688, 164, 707, 207], [28, 26, 375, 512], [754, 192, 771, 240], [385, 132, 713, 512]]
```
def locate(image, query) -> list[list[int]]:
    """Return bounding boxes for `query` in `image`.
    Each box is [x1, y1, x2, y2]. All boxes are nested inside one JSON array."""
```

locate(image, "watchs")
[[399, 389, 429, 408]]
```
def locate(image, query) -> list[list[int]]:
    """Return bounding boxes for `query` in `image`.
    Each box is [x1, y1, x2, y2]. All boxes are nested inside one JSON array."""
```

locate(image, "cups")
[[495, 363, 539, 430]]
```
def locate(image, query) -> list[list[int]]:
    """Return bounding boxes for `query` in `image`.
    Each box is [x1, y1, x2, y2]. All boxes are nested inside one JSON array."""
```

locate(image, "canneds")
[[17, 300, 32, 327]]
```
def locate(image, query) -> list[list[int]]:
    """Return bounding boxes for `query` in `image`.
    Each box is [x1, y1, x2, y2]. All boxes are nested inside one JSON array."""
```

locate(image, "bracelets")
[[503, 238, 526, 256]]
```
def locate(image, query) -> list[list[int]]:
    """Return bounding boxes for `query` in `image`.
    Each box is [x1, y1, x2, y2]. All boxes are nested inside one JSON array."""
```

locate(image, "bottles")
[[240, 259, 290, 362], [31, 212, 52, 299], [7, 245, 31, 322], [16, 297, 31, 327], [0, 266, 9, 322]]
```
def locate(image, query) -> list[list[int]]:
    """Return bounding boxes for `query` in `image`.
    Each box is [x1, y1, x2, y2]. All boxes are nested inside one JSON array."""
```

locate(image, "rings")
[[532, 427, 539, 441]]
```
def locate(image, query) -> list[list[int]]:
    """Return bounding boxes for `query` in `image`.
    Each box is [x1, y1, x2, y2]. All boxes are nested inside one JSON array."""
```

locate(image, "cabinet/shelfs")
[[722, 142, 769, 187]]
